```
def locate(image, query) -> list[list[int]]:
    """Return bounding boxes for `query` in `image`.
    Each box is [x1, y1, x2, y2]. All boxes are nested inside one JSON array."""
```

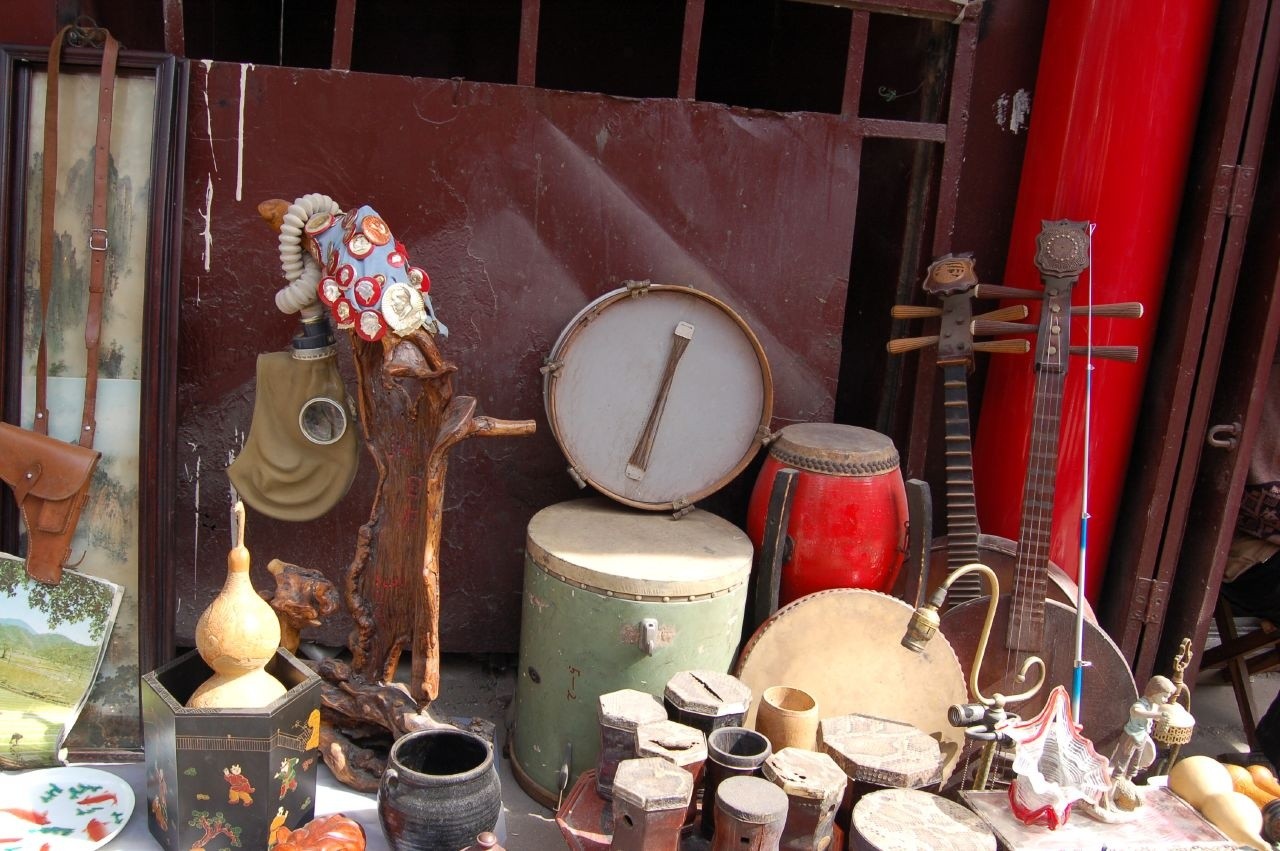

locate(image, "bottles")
[[376, 728, 501, 851]]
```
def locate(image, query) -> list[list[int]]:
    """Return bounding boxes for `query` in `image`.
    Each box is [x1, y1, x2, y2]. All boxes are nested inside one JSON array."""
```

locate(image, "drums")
[[542, 278, 777, 515], [504, 497, 762, 817], [744, 419, 906, 627], [730, 588, 968, 794]]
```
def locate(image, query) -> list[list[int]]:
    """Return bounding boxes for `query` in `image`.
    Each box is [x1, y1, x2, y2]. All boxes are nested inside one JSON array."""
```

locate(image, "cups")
[[700, 727, 772, 842], [755, 685, 819, 753]]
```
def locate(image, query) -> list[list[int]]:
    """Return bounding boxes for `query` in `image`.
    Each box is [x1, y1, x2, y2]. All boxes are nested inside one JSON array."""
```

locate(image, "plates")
[[0, 766, 136, 851]]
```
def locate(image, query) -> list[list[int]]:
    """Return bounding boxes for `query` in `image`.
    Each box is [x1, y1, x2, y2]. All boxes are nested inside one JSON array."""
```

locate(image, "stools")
[[1200, 596, 1280, 754]]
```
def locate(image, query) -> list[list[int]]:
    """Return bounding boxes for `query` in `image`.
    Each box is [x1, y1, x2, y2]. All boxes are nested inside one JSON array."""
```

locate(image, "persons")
[[1108, 675, 1178, 812], [1219, 347, 1280, 778]]
